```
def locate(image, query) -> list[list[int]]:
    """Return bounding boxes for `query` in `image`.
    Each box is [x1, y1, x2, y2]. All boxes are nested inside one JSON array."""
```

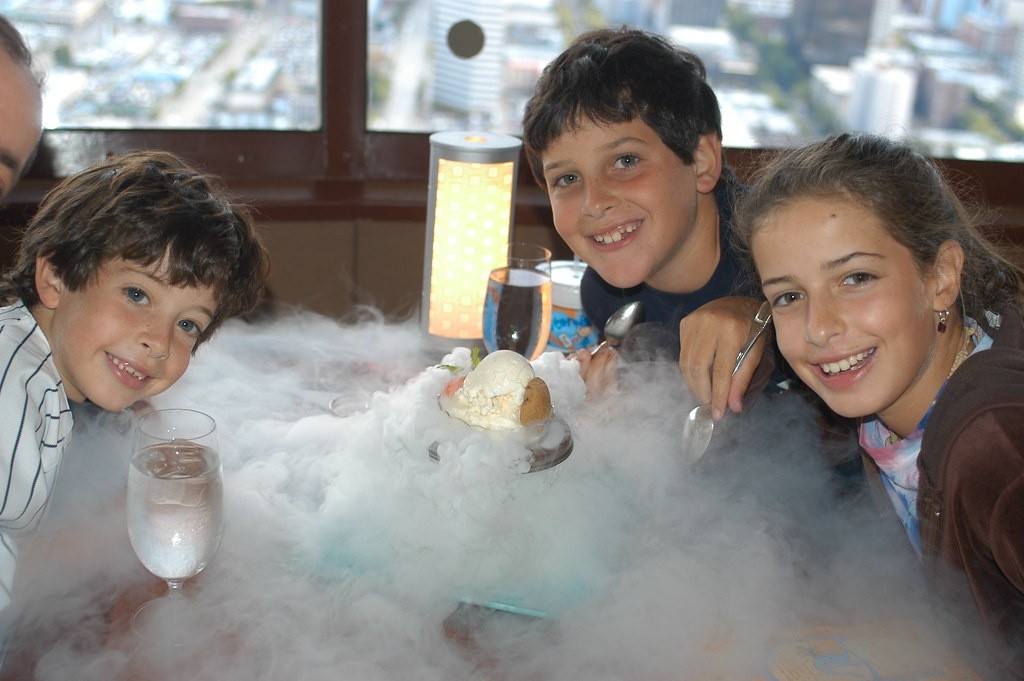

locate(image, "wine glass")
[[126, 408, 226, 645]]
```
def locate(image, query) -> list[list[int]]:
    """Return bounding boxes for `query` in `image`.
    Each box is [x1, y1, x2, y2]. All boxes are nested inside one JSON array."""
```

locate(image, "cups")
[[481, 241, 552, 362], [537, 259, 598, 354]]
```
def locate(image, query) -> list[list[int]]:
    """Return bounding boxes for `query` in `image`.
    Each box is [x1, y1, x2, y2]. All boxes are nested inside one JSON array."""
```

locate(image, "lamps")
[[420, 130, 522, 353]]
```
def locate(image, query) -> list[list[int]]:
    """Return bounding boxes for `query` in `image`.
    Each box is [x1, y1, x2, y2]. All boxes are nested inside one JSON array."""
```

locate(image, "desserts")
[[437, 346, 552, 433]]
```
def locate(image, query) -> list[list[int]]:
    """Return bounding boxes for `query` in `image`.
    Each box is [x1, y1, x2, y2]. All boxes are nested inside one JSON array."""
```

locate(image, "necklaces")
[[885, 326, 980, 451]]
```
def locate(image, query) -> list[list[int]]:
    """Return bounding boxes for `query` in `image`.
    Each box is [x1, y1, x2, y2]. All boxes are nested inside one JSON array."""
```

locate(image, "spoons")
[[681, 301, 772, 465], [590, 301, 646, 361]]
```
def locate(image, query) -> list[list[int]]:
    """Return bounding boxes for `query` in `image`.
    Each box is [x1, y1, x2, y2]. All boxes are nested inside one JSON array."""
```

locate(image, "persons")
[[0, 148, 270, 612], [679, 133, 1024, 681], [0, 17, 44, 203], [522, 25, 794, 398]]
